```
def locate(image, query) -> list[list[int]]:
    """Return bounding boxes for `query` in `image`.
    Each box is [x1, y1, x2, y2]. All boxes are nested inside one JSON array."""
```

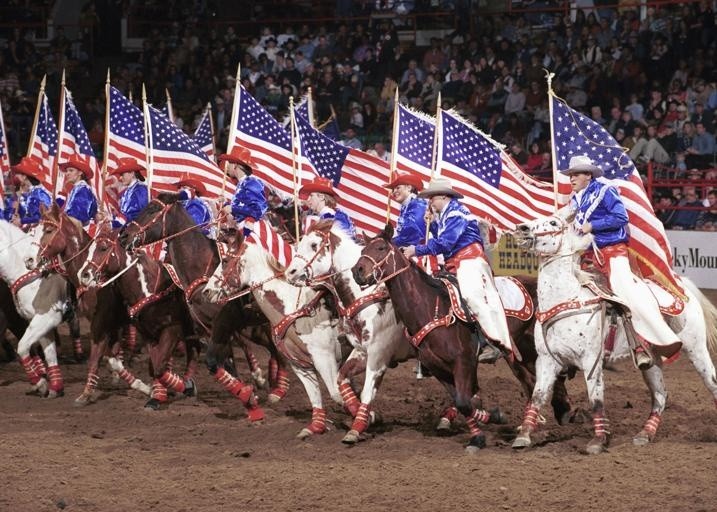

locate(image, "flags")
[[547, 89, 688, 316]]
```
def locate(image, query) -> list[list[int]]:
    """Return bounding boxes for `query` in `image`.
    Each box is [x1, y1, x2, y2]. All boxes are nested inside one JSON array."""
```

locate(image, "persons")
[[403, 177, 525, 363], [559, 156, 684, 370], [0, 3, 716, 247], [218, 145, 267, 315]]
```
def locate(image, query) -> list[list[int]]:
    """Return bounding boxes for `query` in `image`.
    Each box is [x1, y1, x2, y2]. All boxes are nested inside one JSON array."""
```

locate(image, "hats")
[[111, 158, 144, 173], [299, 176, 340, 201], [9, 158, 45, 183], [171, 172, 207, 193], [382, 170, 424, 193], [59, 153, 94, 181], [218, 146, 257, 171], [560, 155, 603, 178]]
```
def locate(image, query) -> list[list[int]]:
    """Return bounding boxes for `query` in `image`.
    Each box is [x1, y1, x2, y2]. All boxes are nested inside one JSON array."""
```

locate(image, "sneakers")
[[478, 348, 503, 362], [637, 350, 650, 369]]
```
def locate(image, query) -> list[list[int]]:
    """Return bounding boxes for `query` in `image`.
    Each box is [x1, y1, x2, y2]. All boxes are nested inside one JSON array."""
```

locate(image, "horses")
[[199, 226, 361, 438], [0, 218, 137, 399], [117, 191, 291, 423], [350, 220, 593, 457], [77, 217, 267, 411], [284, 215, 482, 443], [511, 212, 716, 455], [23, 199, 197, 404]]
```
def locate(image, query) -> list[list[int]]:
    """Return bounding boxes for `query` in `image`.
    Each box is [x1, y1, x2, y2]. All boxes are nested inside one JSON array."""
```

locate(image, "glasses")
[[416, 176, 463, 198]]
